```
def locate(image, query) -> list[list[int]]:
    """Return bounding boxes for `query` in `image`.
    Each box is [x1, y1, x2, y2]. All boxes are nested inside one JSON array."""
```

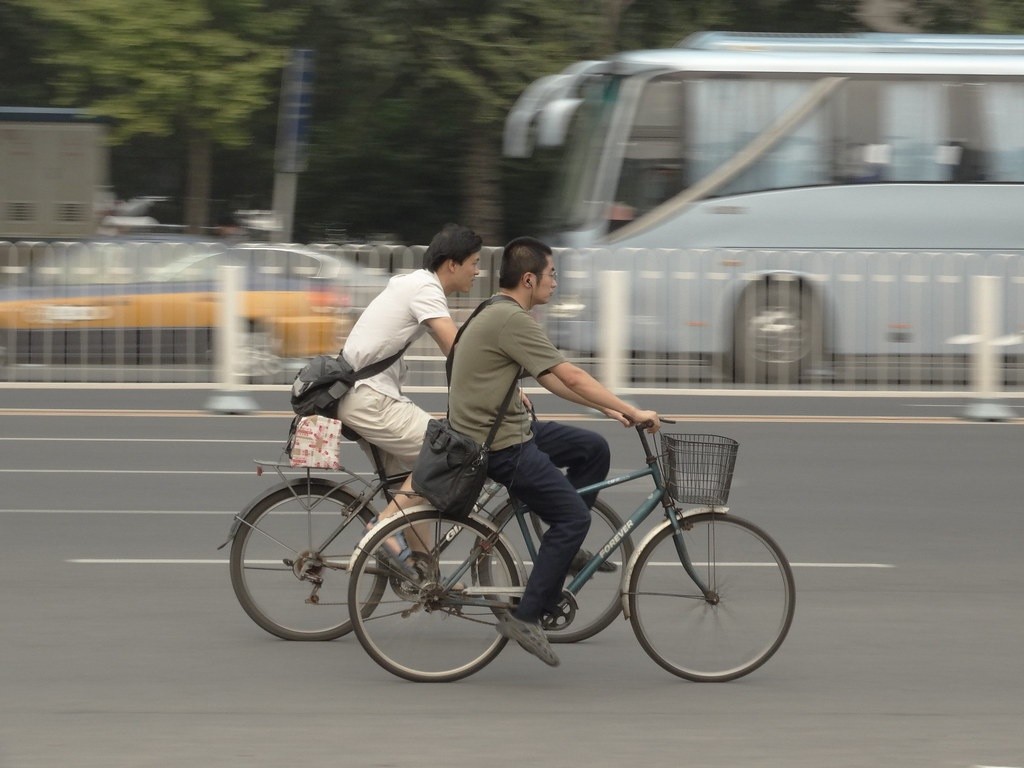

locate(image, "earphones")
[[526, 279, 532, 286]]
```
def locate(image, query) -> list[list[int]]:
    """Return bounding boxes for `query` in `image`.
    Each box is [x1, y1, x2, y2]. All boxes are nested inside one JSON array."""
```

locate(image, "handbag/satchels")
[[413, 419, 489, 523], [291, 355, 353, 416]]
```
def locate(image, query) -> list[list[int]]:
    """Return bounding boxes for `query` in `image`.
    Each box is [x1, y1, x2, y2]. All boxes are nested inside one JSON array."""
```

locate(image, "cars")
[[0, 242, 358, 366], [150, 240, 391, 314]]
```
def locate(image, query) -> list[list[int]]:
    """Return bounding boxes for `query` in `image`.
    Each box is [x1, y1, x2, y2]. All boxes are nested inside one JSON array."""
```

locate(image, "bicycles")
[[347, 417, 797, 684], [214, 402, 636, 646]]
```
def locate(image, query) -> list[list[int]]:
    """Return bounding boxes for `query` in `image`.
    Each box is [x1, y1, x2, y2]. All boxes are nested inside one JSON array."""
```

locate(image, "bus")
[[503, 33, 1024, 388]]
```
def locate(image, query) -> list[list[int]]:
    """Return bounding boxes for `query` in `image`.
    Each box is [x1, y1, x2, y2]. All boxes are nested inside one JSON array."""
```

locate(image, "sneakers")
[[496, 610, 560, 667], [568, 549, 617, 571]]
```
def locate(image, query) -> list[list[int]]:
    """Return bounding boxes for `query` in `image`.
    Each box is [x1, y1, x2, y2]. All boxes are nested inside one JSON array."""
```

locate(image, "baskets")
[[662, 433, 740, 506]]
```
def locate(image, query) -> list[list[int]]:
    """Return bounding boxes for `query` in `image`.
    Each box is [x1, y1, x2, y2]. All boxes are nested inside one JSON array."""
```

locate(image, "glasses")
[[521, 270, 557, 281]]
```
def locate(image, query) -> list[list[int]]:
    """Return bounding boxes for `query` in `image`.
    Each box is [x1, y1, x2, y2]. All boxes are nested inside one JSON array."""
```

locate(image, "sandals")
[[363, 517, 420, 581]]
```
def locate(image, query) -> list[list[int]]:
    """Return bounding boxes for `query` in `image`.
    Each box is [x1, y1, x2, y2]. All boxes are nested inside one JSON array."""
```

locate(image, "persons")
[[448, 237, 660, 667], [337, 225, 483, 590]]
